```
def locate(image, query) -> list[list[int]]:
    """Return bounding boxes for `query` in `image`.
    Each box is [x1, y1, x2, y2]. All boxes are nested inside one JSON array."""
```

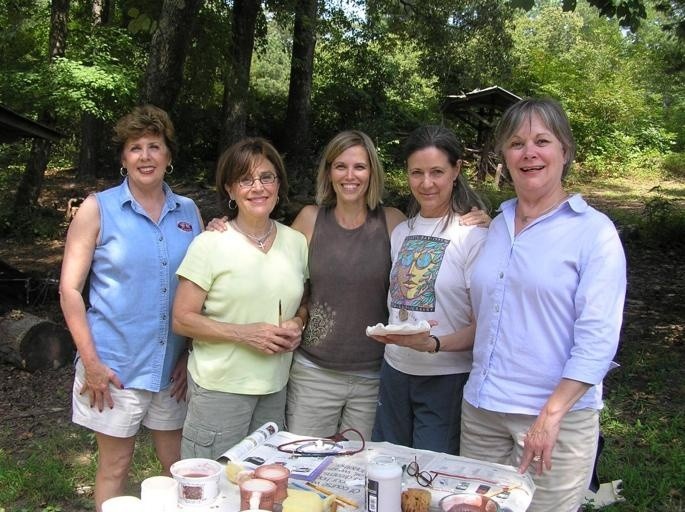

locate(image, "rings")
[[532, 455, 543, 462]]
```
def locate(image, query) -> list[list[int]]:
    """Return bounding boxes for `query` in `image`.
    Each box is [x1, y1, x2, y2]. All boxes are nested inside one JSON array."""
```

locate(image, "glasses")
[[406, 455, 439, 489], [234, 172, 279, 188]]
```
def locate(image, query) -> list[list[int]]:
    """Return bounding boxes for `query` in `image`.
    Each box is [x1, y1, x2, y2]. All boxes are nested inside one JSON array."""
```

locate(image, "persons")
[[170, 135, 311, 458], [368, 122, 488, 455], [460, 97, 629, 511], [206, 130, 491, 442], [57, 104, 207, 512]]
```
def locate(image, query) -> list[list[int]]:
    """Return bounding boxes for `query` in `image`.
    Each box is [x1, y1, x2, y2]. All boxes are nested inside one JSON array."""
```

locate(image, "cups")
[[239, 479, 277, 512], [141, 475, 180, 512], [237, 464, 289, 504]]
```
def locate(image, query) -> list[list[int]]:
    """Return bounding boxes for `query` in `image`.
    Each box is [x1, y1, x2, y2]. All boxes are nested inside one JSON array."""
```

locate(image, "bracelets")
[[428, 334, 441, 354]]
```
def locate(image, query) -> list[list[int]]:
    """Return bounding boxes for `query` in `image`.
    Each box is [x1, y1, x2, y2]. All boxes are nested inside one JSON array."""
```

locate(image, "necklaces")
[[397, 209, 448, 320], [231, 215, 275, 252]]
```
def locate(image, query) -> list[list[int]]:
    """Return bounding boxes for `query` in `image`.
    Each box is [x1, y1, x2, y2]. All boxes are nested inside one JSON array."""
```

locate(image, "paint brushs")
[[291, 481, 359, 508], [279, 299, 281, 362]]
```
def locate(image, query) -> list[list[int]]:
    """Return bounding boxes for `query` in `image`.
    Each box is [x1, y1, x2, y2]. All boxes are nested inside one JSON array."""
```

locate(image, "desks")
[[218, 420, 536, 512]]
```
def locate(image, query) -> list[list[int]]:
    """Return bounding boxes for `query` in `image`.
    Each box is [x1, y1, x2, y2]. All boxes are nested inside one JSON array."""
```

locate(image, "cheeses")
[[282, 489, 322, 512], [224, 464, 245, 483]]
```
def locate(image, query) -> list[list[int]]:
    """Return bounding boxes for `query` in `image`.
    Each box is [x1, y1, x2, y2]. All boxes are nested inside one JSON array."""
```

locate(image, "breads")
[[400, 487, 432, 512]]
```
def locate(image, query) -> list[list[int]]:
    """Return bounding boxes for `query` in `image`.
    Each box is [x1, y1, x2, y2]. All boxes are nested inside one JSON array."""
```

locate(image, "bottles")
[[367, 455, 402, 512]]
